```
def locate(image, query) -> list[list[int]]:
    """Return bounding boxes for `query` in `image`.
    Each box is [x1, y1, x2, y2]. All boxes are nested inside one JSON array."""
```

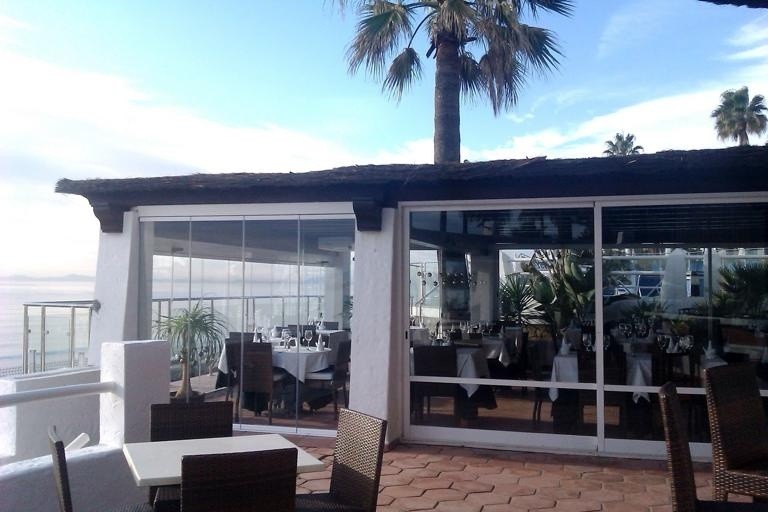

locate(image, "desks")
[[122, 434, 324, 487], [410, 326, 523, 420]]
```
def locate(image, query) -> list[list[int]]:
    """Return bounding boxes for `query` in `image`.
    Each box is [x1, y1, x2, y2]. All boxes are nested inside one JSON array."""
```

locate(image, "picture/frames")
[[122, 434, 324, 487], [410, 326, 523, 420]]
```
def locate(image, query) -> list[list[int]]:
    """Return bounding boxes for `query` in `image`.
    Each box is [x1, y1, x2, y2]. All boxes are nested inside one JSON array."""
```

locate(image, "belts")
[[304, 330, 313, 351], [460, 320, 493, 337], [617, 318, 696, 353]]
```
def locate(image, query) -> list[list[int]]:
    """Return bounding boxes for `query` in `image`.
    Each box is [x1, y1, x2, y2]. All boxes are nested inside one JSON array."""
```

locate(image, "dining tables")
[[150, 292, 235, 403]]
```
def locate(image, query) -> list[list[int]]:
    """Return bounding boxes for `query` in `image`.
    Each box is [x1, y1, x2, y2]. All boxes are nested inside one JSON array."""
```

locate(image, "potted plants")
[[150, 292, 235, 403]]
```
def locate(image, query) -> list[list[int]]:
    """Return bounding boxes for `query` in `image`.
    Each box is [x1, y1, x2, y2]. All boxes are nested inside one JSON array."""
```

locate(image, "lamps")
[[226, 322, 351, 425], [520, 332, 528, 390], [149, 402, 233, 506], [180, 448, 297, 512], [296, 408, 387, 512], [48, 425, 153, 512]]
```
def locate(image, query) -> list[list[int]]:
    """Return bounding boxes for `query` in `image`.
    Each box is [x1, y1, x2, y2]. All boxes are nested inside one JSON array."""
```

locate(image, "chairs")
[[520, 332, 528, 390], [296, 408, 387, 512], [149, 402, 233, 506], [226, 322, 351, 425], [48, 425, 153, 512], [656, 379, 767, 512], [700, 364, 767, 504], [531, 319, 768, 440], [180, 448, 297, 512]]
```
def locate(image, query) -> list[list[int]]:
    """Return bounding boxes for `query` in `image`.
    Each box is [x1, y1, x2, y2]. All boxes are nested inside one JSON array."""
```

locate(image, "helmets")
[[309, 347, 333, 353]]
